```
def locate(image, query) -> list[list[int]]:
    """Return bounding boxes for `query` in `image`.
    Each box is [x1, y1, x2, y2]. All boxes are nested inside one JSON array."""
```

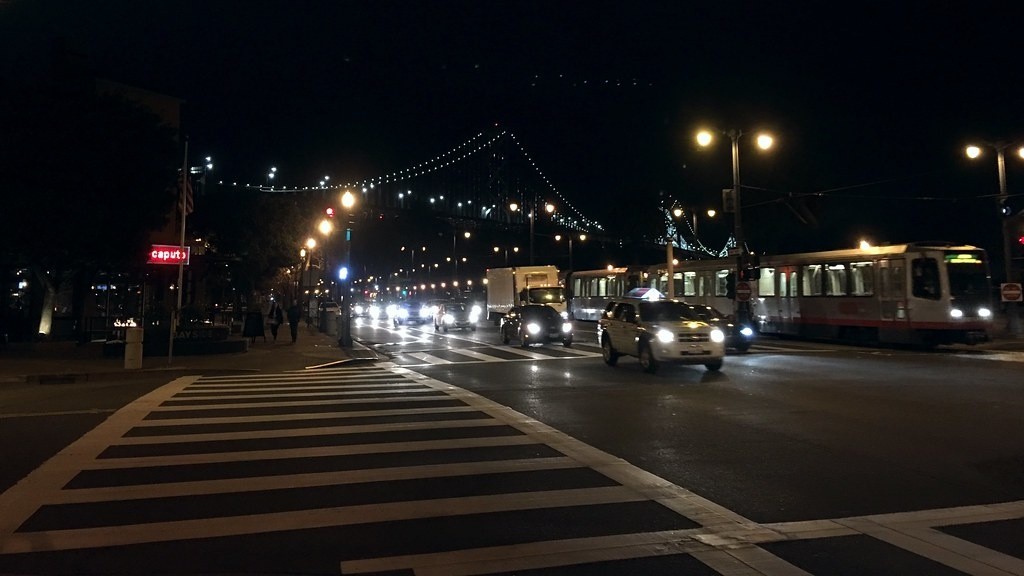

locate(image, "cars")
[[319, 297, 483, 333], [688, 303, 755, 352], [500, 305, 575, 347]]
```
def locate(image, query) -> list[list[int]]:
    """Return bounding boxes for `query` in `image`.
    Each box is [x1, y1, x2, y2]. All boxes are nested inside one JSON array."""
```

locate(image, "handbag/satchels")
[[266, 315, 279, 325]]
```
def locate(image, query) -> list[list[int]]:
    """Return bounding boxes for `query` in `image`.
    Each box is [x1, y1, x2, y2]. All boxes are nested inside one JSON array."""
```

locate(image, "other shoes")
[[274, 336, 277, 340], [251, 340, 255, 343]]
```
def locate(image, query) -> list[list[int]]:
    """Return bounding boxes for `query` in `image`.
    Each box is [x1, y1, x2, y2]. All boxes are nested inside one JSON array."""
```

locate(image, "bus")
[[641, 243, 994, 346], [567, 269, 643, 321]]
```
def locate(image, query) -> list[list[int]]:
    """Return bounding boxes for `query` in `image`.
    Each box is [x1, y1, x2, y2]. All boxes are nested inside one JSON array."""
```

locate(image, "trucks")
[[486, 266, 571, 326]]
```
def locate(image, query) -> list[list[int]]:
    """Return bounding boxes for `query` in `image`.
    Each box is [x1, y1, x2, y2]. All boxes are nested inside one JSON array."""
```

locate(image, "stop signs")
[[1001, 282, 1023, 302]]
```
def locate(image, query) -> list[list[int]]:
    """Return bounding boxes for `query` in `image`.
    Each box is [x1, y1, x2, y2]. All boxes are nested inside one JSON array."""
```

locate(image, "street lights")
[[361, 231, 519, 293], [283, 191, 356, 346], [964, 140, 1024, 337], [555, 232, 586, 266], [508, 202, 554, 265], [696, 128, 774, 250]]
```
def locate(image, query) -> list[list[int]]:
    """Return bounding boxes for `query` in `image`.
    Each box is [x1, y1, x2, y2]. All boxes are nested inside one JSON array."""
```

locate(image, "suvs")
[[596, 298, 725, 373]]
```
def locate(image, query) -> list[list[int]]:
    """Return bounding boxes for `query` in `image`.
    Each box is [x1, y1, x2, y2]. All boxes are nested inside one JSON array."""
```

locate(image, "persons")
[[268, 302, 284, 344], [914, 266, 931, 299], [287, 299, 301, 345]]
